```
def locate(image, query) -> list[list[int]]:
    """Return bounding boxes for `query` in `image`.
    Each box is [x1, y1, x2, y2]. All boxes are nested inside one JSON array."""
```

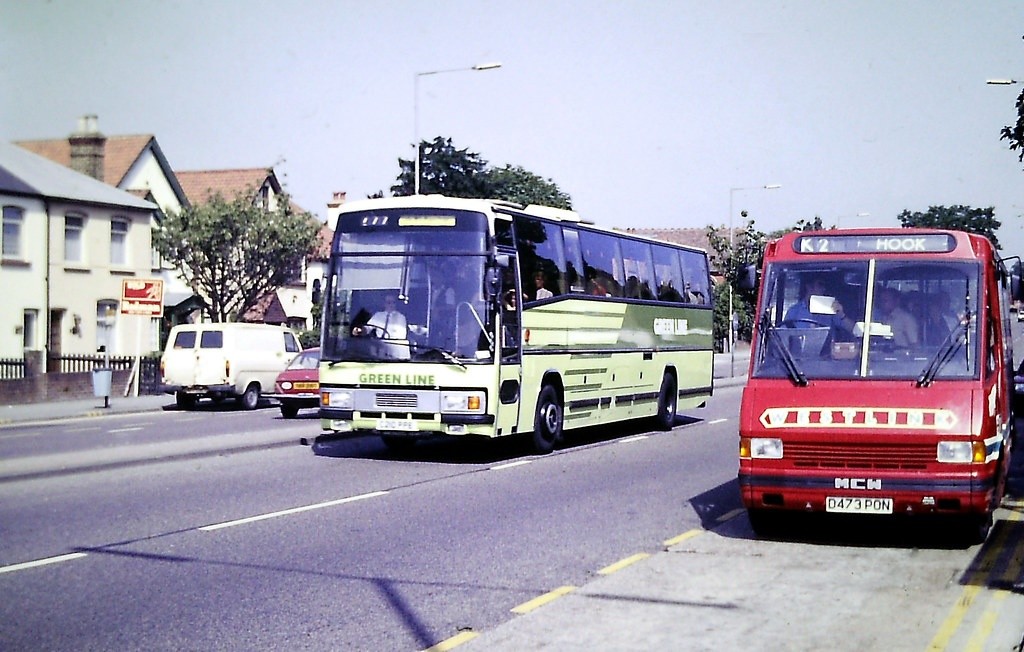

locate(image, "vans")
[[158, 322, 303, 410]]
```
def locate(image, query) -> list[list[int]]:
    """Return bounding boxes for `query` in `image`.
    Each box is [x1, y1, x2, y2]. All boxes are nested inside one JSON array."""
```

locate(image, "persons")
[[780, 278, 973, 351], [351, 294, 408, 361], [431, 269, 704, 311]]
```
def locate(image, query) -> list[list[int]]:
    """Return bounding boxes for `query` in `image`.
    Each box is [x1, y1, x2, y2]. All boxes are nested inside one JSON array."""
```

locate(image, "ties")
[[382, 315, 390, 338]]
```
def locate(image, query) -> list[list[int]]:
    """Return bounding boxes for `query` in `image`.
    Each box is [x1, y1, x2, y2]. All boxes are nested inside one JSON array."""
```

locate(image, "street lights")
[[729, 185, 782, 355], [413, 63, 501, 194]]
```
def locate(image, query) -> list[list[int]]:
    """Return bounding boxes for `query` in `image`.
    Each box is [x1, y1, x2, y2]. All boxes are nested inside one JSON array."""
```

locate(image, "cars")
[[1012, 358, 1024, 411], [274, 347, 321, 418], [1017, 310, 1024, 322]]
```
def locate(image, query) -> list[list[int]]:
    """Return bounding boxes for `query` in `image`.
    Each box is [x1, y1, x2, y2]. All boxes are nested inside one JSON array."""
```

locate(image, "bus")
[[316, 195, 713, 454], [738, 227, 1013, 544]]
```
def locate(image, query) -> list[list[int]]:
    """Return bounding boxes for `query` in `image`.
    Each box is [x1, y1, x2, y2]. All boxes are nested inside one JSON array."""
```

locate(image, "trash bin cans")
[[92, 367, 113, 398]]
